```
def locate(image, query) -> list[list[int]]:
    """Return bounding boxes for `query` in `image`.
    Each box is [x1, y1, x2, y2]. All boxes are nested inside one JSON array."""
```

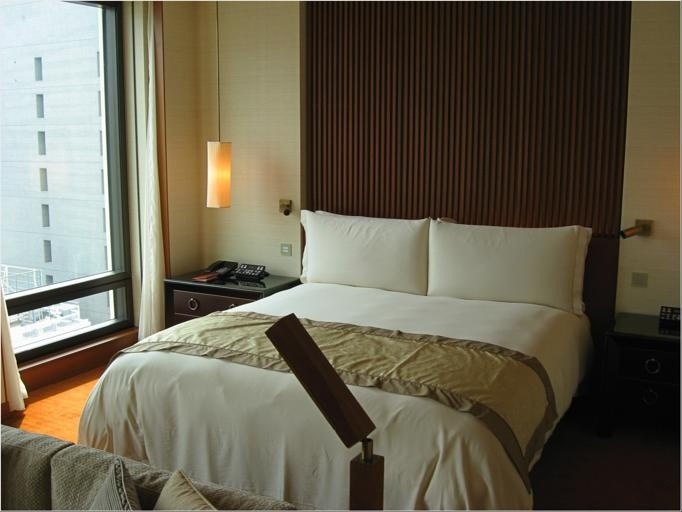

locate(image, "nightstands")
[[163, 268, 300, 327], [608, 311, 680, 433]]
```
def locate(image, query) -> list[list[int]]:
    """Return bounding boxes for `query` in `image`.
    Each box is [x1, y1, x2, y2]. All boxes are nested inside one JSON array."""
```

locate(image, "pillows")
[[300, 210, 430, 295], [427, 216, 592, 316]]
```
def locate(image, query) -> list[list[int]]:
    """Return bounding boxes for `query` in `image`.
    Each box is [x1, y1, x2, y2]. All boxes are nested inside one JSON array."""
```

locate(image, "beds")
[[79, 209, 593, 510]]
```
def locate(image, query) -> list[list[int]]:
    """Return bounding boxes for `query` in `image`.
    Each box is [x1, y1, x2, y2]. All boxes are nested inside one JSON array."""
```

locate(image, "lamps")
[[205, 2, 233, 208]]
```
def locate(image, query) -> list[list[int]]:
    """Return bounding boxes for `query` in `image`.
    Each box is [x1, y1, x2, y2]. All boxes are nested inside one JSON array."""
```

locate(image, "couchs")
[[2, 422, 295, 510]]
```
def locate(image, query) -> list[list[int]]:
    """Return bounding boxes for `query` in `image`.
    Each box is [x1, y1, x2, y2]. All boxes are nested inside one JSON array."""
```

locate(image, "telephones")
[[204, 260, 238, 276]]
